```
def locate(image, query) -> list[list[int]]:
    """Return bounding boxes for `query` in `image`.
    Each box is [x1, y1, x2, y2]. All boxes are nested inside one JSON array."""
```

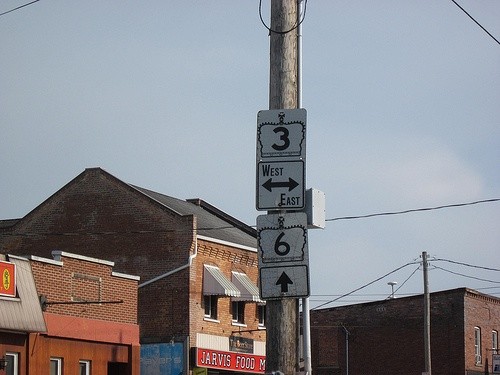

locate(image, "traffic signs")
[[255, 107, 307, 212], [256, 212, 311, 300]]
